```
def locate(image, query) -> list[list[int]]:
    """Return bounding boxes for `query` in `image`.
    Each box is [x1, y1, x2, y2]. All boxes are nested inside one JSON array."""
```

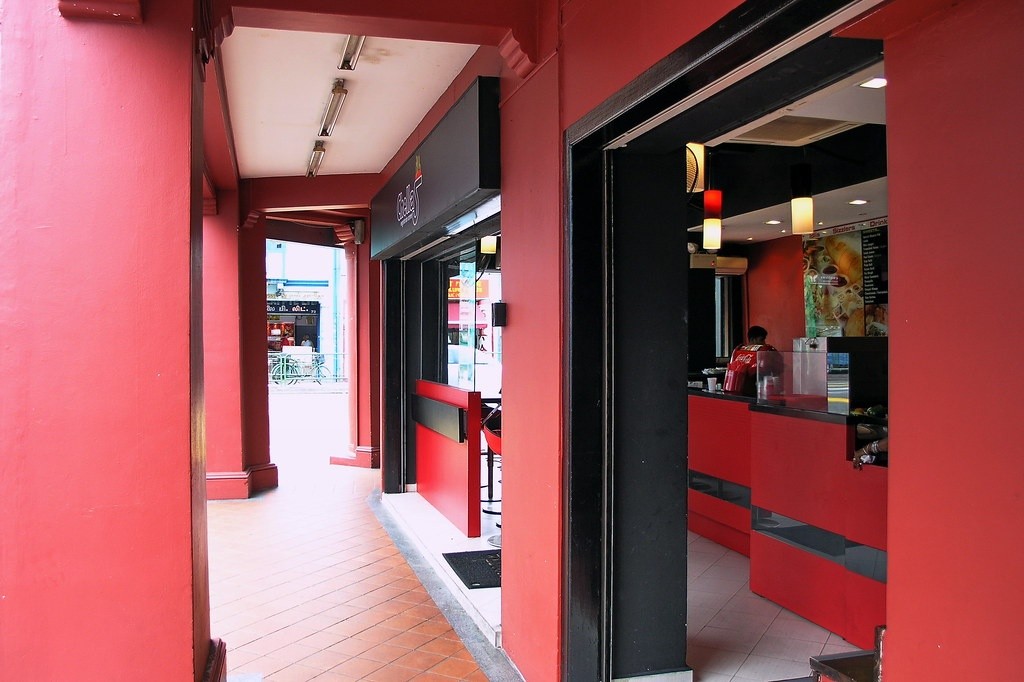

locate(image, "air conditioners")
[[715, 257, 748, 275]]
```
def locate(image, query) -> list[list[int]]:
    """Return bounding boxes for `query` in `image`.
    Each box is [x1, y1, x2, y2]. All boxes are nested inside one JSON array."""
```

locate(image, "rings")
[[860, 463, 862, 465], [853, 457, 856, 461]]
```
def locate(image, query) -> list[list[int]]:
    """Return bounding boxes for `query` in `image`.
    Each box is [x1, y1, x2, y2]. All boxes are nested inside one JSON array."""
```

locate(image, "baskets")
[[316, 354, 325, 365]]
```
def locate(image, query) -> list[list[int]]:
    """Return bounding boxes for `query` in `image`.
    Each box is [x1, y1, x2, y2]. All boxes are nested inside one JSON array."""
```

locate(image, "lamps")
[[790, 197, 814, 235], [338, 33, 367, 72], [305, 139, 326, 179], [480, 235, 496, 254], [317, 79, 348, 137], [703, 164, 723, 219], [703, 217, 721, 249], [686, 141, 705, 193]]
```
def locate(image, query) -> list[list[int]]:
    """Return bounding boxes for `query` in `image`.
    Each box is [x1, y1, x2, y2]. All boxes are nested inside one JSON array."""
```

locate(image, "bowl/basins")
[[821, 264, 839, 275], [829, 274, 850, 291], [818, 256, 831, 268], [817, 245, 825, 255]]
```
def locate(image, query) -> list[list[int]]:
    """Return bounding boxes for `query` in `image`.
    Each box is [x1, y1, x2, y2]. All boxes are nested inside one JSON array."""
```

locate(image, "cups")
[[707, 378, 717, 391], [758, 376, 784, 400]]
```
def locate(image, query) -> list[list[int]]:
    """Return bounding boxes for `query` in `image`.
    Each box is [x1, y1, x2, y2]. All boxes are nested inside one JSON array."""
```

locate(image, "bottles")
[[867, 405, 883, 416]]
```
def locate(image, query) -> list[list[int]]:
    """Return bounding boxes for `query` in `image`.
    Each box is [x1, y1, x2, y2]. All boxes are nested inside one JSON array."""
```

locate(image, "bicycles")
[[267, 353, 332, 385]]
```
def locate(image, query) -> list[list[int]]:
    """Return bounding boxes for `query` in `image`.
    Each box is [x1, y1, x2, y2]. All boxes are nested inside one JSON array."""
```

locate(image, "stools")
[[481, 406, 501, 502], [483, 416, 501, 548]]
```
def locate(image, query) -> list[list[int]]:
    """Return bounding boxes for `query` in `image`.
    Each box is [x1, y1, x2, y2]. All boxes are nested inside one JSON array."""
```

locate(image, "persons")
[[281, 332, 314, 352], [853, 437, 888, 470], [746, 326, 768, 344]]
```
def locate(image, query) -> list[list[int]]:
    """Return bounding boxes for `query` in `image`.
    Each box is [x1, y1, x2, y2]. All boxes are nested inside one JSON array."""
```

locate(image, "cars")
[[446, 344, 503, 401]]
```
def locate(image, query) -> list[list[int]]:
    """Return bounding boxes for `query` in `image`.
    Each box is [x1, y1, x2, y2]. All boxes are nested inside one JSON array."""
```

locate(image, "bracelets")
[[863, 440, 880, 455]]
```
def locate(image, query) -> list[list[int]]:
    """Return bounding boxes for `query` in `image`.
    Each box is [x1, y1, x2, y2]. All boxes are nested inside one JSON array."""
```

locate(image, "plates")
[[828, 283, 864, 327]]
